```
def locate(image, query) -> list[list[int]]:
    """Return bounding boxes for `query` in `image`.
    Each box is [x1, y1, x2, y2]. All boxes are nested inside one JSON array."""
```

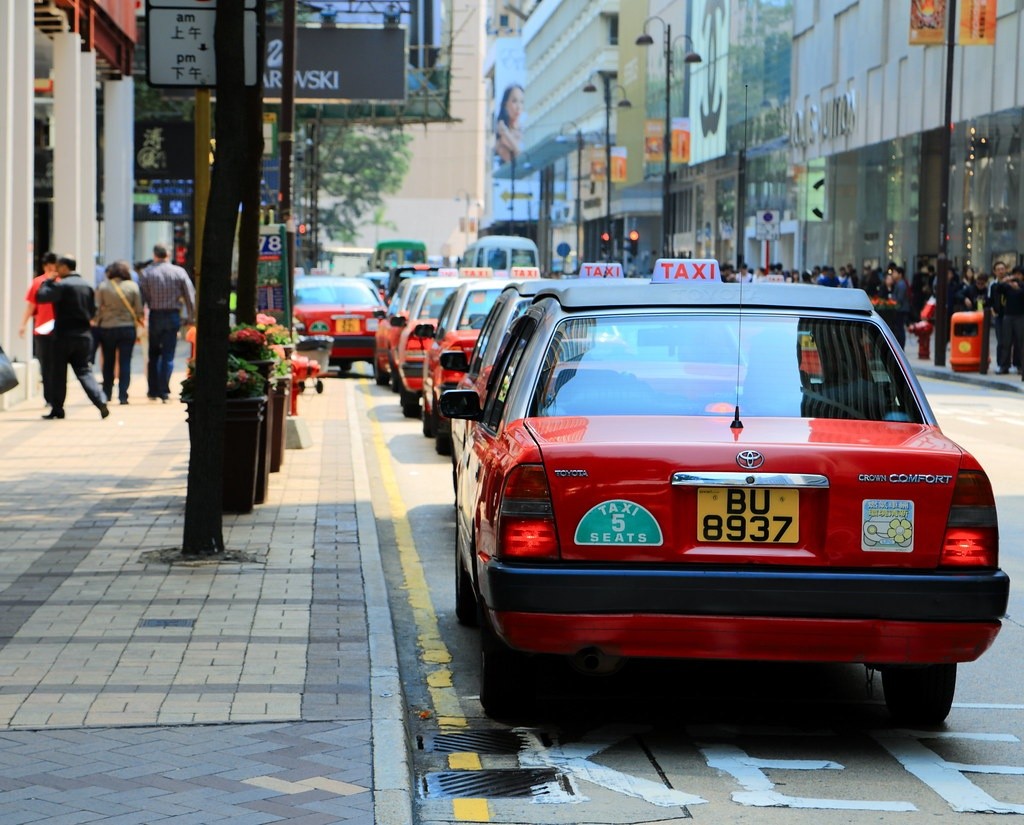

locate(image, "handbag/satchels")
[[0, 346, 19, 394], [134, 317, 146, 344]]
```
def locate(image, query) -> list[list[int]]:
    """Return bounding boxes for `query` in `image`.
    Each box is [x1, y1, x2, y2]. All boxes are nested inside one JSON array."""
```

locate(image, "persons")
[[35, 254, 110, 420], [90, 260, 154, 405], [138, 244, 196, 403], [719, 261, 1024, 381], [20, 252, 61, 407], [496, 83, 524, 164], [542, 270, 563, 279]]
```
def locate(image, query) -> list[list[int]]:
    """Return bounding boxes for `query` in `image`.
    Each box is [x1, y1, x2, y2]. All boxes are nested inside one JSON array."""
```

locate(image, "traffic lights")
[[600, 232, 609, 253], [629, 231, 639, 257]]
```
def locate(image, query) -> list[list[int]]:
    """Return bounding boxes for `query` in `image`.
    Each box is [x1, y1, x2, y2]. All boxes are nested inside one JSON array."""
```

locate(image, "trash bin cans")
[[185, 327, 197, 378], [949, 311, 991, 372]]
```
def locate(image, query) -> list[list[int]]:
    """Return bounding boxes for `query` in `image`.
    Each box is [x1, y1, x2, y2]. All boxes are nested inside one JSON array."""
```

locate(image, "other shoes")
[[100, 404, 109, 418], [996, 363, 1011, 374], [1017, 366, 1024, 374], [42, 407, 65, 419]]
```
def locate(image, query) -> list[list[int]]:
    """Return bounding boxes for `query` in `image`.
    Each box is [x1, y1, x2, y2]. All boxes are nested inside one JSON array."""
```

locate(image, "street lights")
[[583, 70, 632, 263], [556, 120, 607, 274], [634, 16, 703, 258], [492, 150, 532, 236]]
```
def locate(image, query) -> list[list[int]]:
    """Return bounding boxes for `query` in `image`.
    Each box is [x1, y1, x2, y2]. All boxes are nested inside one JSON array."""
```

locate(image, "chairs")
[[429, 303, 443, 318], [468, 313, 486, 329], [555, 369, 634, 410]]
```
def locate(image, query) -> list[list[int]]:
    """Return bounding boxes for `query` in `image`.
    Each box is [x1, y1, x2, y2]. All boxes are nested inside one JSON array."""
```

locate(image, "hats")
[[827, 267, 835, 274], [42, 252, 56, 264]]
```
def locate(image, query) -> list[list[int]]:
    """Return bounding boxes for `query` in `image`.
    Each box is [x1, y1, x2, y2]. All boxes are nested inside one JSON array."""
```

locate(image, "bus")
[[460, 236, 542, 270], [366, 240, 428, 272]]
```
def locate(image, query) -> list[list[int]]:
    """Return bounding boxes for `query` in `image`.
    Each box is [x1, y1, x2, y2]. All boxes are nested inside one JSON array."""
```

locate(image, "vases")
[[185, 345, 295, 515]]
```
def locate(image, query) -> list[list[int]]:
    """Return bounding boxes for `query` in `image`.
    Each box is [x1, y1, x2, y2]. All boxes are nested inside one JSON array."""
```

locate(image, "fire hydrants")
[[908, 319, 933, 358]]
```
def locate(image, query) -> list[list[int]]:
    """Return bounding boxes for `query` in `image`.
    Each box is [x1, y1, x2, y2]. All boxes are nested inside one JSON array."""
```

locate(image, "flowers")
[[179, 312, 293, 397]]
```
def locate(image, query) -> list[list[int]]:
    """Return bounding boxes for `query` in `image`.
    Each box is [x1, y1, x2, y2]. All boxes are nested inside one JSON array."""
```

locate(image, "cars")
[[437, 258, 1012, 731], [291, 258, 747, 453]]
